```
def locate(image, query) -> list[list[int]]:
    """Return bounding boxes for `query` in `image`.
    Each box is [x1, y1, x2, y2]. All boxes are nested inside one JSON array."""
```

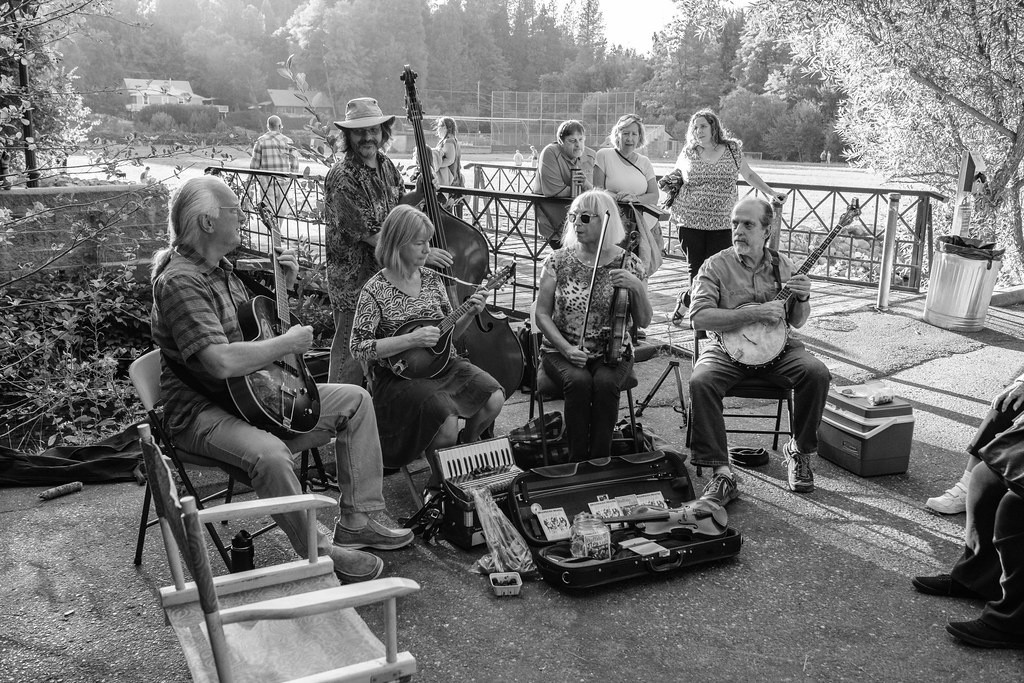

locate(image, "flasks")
[[571, 168, 582, 197]]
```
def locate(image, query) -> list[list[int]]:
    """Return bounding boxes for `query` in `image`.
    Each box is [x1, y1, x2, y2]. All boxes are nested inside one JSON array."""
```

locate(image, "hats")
[[334, 97, 396, 133]]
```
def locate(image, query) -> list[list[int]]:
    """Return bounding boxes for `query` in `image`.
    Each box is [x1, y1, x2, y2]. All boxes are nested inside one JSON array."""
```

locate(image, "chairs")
[[685, 289, 795, 476], [529, 299, 639, 466], [137, 422, 421, 683], [128, 348, 341, 572]]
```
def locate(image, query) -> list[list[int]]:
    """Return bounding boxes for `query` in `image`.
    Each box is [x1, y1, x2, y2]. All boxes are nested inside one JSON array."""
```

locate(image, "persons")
[[535, 189, 653, 464], [672, 110, 788, 327], [414, 118, 462, 219], [348, 204, 505, 506], [532, 120, 597, 249], [140, 167, 152, 182], [528, 146, 538, 175], [689, 195, 832, 506], [247, 115, 299, 241], [820, 150, 827, 164], [152, 177, 414, 583], [323, 98, 440, 384], [594, 114, 659, 336], [912, 373, 1024, 649], [514, 150, 523, 165], [827, 151, 831, 163]]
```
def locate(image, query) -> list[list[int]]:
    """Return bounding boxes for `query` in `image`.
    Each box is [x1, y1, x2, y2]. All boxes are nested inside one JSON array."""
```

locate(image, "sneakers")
[[926, 478, 966, 514], [697, 474, 741, 506], [783, 439, 814, 493]]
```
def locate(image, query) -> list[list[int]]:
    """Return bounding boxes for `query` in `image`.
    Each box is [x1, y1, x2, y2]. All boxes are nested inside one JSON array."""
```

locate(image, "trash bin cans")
[[922, 236, 1002, 332]]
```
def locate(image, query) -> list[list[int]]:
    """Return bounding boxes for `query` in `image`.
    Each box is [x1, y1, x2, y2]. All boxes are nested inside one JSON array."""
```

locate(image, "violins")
[[605, 231, 639, 364], [597, 500, 728, 540]]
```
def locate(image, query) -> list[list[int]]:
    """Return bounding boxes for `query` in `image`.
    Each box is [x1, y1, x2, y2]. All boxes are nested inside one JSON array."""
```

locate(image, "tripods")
[[634, 361, 687, 429]]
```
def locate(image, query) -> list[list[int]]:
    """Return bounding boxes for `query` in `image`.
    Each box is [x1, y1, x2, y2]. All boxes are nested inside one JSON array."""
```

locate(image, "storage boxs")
[[815, 383, 915, 476]]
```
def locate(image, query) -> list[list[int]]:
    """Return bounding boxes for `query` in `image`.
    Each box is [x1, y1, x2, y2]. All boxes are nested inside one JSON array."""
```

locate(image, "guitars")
[[215, 203, 322, 437], [719, 197, 862, 369]]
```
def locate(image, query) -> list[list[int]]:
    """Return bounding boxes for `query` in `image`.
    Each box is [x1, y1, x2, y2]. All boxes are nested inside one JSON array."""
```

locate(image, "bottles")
[[571, 520, 611, 560], [231, 529, 254, 573]]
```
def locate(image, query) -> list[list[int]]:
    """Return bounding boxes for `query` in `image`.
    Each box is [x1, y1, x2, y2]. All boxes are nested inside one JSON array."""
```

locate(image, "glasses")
[[618, 114, 642, 128], [568, 212, 600, 225], [351, 126, 383, 136], [219, 206, 243, 215]]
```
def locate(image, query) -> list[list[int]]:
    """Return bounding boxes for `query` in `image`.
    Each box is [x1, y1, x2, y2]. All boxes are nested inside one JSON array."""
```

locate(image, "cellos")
[[401, 65, 524, 396]]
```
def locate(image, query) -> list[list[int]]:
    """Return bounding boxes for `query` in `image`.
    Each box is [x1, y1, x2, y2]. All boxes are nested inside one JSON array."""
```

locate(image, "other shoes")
[[331, 515, 414, 551], [912, 574, 975, 598], [322, 544, 383, 584], [945, 619, 1024, 649]]
